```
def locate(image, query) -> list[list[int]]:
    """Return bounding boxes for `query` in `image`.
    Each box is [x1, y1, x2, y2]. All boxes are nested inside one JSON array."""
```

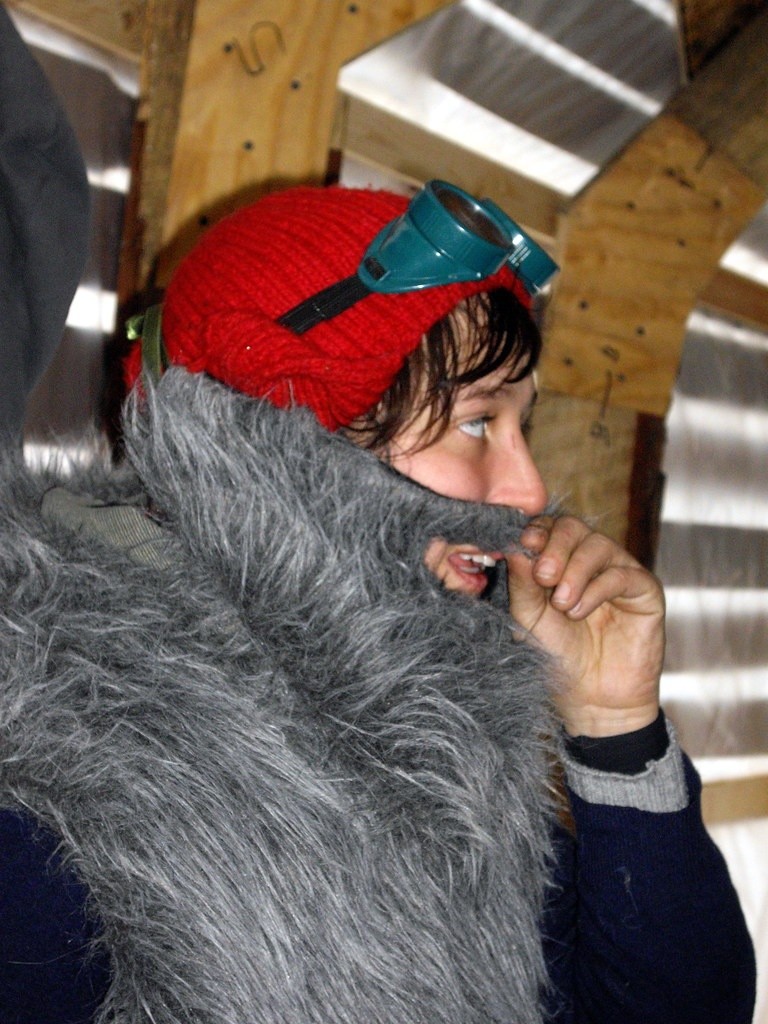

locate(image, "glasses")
[[359, 180, 562, 295]]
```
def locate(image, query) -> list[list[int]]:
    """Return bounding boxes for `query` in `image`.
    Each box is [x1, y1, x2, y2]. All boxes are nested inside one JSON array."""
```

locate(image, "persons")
[[0, 181, 759, 1024]]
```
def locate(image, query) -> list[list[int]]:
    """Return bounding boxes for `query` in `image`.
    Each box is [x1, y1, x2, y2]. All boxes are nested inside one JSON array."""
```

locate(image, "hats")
[[125, 187, 534, 433]]
